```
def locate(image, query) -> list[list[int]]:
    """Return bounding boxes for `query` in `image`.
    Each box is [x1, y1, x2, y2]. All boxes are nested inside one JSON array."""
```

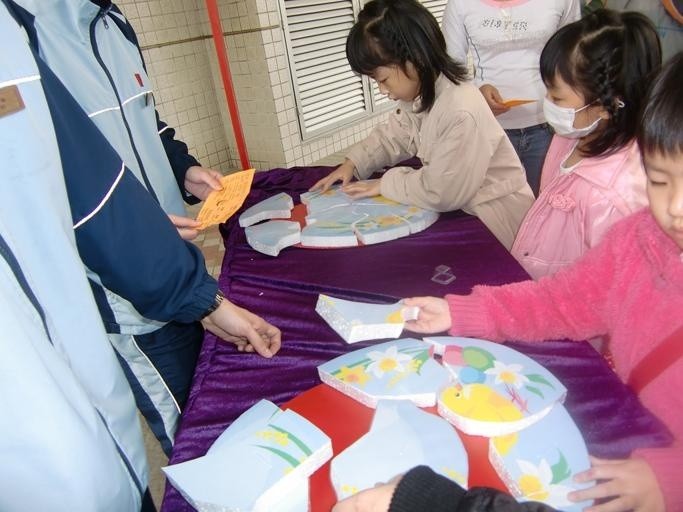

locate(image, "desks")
[[157, 163, 682, 511]]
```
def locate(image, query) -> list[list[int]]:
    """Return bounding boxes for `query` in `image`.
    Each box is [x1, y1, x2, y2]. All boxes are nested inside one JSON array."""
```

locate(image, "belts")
[[506, 124, 548, 135]]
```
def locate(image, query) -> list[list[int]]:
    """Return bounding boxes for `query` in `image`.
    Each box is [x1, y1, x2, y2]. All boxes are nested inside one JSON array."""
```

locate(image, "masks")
[[543, 98, 626, 139]]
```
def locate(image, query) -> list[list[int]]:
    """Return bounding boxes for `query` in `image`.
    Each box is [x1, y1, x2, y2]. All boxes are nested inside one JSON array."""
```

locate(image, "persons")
[[328, 466, 562, 510], [14, 0, 228, 460], [657, 2, 683, 42], [507, 10, 662, 280], [393, 52, 682, 510], [1, 0, 283, 509], [441, 0, 581, 200], [307, 0, 535, 251]]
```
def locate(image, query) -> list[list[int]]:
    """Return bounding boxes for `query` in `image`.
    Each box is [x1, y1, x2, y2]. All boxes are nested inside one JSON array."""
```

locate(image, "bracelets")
[[198, 293, 223, 318]]
